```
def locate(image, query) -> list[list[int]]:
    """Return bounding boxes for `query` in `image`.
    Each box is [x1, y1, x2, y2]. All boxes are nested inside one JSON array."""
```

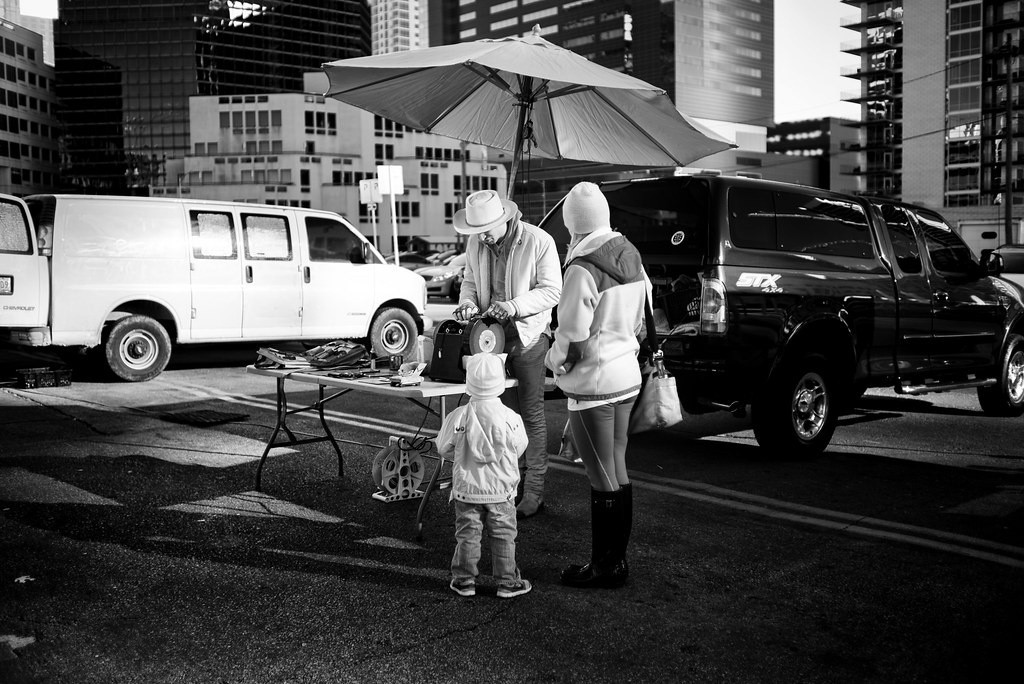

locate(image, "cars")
[[381, 248, 468, 304]]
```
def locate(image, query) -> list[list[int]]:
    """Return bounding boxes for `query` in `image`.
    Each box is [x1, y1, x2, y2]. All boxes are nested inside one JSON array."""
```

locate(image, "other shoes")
[[517, 493, 544, 517]]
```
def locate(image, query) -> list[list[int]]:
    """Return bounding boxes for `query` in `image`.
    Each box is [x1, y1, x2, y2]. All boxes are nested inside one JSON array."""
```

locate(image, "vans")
[[0, 193, 427, 385]]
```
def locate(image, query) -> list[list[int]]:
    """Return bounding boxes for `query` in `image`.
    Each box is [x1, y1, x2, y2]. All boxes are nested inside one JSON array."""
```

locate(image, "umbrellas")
[[323, 23, 739, 203]]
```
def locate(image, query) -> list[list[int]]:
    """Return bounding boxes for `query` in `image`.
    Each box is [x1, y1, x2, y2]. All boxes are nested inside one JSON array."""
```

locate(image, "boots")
[[559, 481, 633, 589]]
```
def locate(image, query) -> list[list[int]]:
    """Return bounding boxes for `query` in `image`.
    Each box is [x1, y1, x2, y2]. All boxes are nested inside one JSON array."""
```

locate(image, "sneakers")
[[497, 580, 532, 597], [450, 579, 475, 596]]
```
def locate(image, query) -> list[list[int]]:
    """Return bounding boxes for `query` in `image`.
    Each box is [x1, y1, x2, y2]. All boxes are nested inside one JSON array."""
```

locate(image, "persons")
[[452, 189, 564, 518], [548, 181, 658, 588], [435, 351, 536, 597]]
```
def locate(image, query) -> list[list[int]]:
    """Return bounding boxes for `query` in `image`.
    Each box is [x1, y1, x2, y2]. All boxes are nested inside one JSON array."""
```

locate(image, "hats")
[[465, 351, 506, 399], [562, 181, 610, 234], [453, 190, 518, 235]]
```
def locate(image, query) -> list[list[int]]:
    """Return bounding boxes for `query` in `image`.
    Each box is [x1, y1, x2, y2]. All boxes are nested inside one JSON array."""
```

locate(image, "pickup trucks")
[[527, 166, 1024, 463]]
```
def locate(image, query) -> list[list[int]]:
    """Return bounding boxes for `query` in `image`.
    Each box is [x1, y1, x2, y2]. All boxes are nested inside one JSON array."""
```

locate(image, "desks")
[[245, 365, 520, 540]]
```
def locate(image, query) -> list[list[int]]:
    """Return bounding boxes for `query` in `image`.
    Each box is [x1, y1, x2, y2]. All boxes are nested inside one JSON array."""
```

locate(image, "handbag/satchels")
[[627, 357, 683, 434]]
